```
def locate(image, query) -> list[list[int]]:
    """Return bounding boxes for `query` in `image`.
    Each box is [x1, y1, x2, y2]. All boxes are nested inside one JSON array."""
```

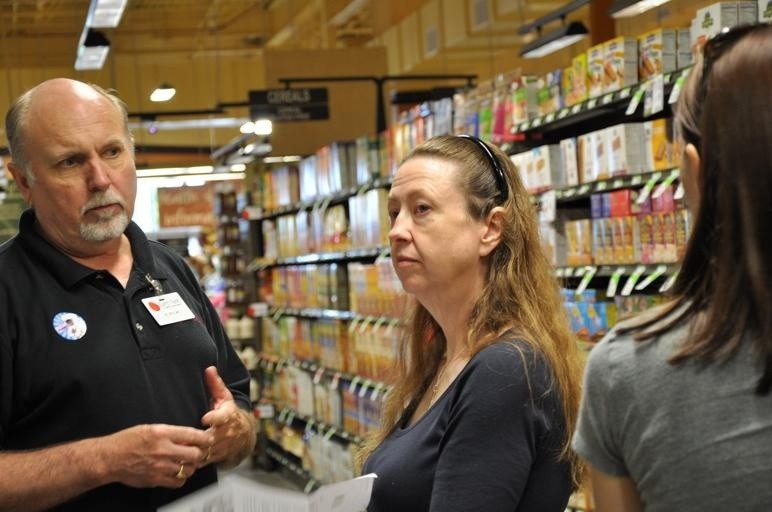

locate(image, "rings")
[[175, 463, 187, 479]]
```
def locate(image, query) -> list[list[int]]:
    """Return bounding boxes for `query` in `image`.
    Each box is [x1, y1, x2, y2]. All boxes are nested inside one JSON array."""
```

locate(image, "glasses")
[[699, 21, 766, 94]]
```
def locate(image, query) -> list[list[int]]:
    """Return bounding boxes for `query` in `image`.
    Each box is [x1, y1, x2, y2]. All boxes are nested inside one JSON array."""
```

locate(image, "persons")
[[356, 131, 585, 512], [0, 76, 257, 512], [570, 23, 768, 511]]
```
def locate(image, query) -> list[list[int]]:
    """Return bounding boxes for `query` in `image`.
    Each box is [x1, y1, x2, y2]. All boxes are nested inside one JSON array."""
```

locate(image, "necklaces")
[[427, 348, 465, 393]]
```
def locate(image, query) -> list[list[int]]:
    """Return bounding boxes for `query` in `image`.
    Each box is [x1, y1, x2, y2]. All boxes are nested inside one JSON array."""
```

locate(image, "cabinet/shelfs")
[[244, 65, 690, 492]]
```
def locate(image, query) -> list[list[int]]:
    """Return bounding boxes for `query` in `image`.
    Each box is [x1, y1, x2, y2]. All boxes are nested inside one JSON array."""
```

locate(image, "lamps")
[[519, 20, 589, 59]]
